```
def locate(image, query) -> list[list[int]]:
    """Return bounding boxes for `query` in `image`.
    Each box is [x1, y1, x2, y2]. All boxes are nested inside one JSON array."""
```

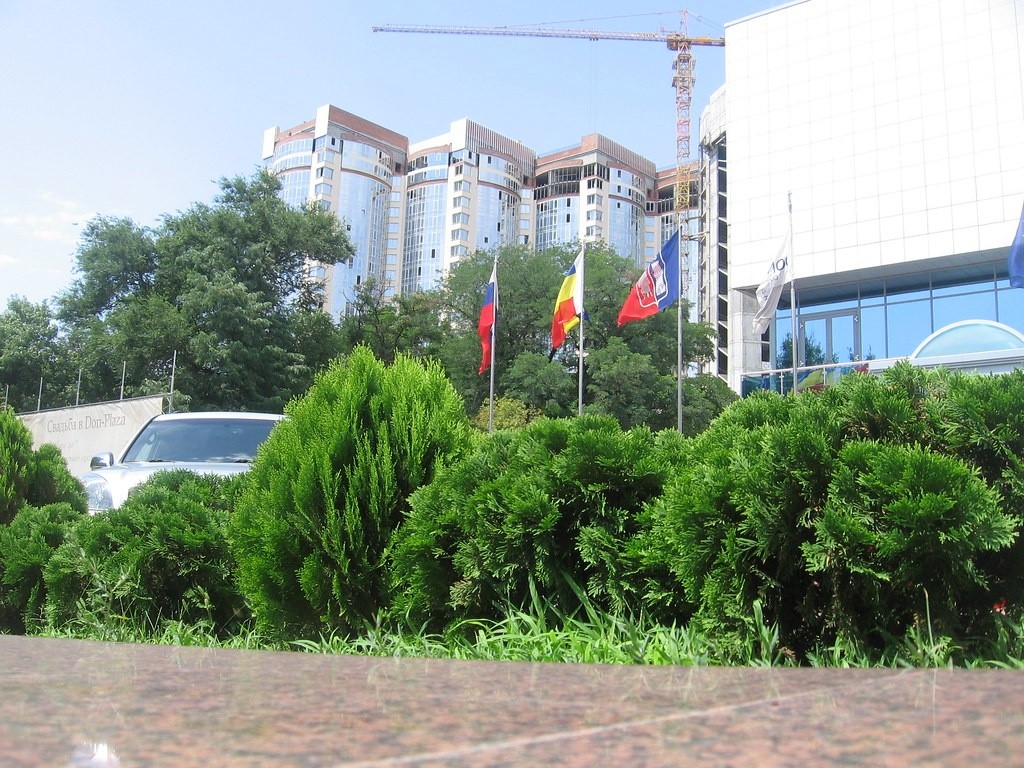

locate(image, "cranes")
[[370, 8, 725, 307]]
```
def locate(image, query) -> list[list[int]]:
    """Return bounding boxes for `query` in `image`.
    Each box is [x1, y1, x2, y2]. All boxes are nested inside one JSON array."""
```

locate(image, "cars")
[[77, 410, 291, 518]]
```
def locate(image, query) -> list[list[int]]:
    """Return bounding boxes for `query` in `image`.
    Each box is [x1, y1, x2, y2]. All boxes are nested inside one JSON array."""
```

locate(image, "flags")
[[617, 226, 682, 330], [478, 262, 500, 374], [551, 247, 590, 350], [748, 214, 792, 335]]
